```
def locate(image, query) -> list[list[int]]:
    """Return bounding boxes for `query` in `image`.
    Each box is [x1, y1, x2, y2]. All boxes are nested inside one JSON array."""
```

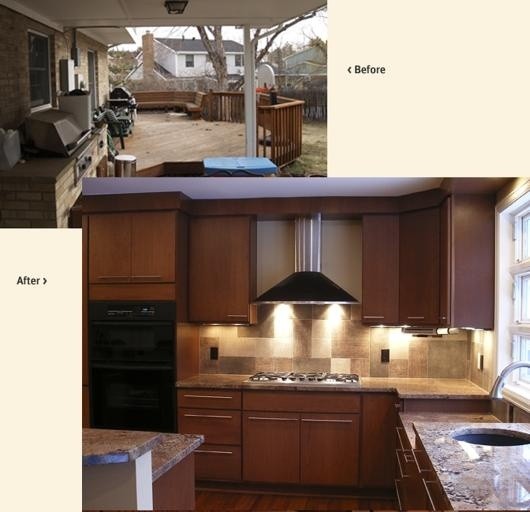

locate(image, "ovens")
[[89, 300, 176, 433]]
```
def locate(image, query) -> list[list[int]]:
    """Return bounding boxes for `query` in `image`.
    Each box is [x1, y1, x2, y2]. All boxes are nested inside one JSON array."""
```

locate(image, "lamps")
[[164, 0, 189, 14]]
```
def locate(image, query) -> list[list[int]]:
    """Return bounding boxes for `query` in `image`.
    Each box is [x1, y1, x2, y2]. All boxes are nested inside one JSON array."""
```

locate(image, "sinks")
[[451, 426, 530, 446]]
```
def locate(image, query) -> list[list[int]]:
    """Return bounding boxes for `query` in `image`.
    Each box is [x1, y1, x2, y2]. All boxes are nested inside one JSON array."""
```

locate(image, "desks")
[[203, 157, 278, 177]]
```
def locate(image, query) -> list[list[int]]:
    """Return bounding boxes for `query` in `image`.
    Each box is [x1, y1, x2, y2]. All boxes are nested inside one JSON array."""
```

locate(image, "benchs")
[[186, 92, 206, 120]]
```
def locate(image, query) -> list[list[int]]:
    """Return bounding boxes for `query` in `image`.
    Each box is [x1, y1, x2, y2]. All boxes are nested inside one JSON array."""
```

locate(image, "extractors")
[[250, 214, 361, 307]]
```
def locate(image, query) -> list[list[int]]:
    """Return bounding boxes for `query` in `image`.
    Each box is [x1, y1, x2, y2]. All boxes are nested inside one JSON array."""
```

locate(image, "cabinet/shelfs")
[[178, 390, 242, 483], [361, 392, 398, 489], [189, 215, 254, 322], [399, 206, 450, 324], [393, 395, 455, 512], [244, 388, 360, 490], [362, 214, 399, 325], [89, 210, 178, 283]]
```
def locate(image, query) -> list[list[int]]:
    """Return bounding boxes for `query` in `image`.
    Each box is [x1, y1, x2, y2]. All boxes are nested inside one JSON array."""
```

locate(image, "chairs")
[[95, 109, 133, 149]]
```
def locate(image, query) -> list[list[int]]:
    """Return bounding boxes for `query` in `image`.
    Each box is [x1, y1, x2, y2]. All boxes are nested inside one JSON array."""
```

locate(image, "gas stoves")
[[245, 370, 361, 388]]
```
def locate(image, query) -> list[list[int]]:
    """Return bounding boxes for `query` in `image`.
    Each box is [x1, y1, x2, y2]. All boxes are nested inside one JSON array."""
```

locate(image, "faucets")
[[489, 361, 530, 400]]
